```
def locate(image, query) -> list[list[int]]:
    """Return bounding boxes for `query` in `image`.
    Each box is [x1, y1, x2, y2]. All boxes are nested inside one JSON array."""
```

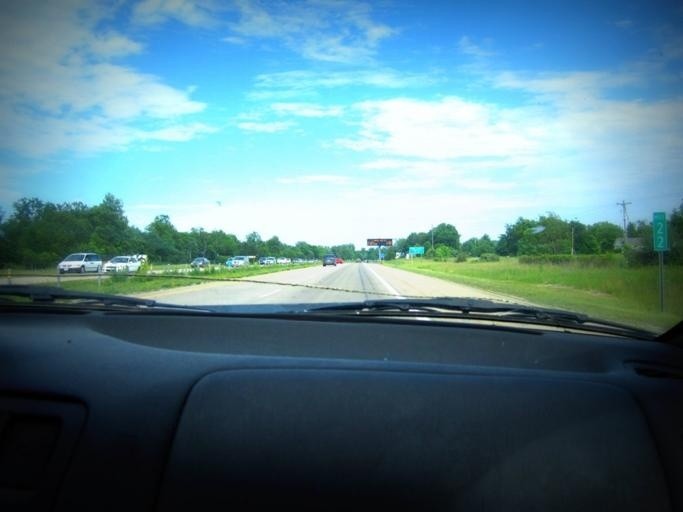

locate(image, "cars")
[[191, 257, 209, 267], [231, 256, 248, 266], [103, 254, 141, 273], [336, 257, 343, 264], [257, 255, 290, 264]]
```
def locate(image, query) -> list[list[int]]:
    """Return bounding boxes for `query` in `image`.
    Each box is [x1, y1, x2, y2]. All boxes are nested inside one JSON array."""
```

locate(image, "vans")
[[58, 252, 102, 273], [323, 254, 336, 266]]
